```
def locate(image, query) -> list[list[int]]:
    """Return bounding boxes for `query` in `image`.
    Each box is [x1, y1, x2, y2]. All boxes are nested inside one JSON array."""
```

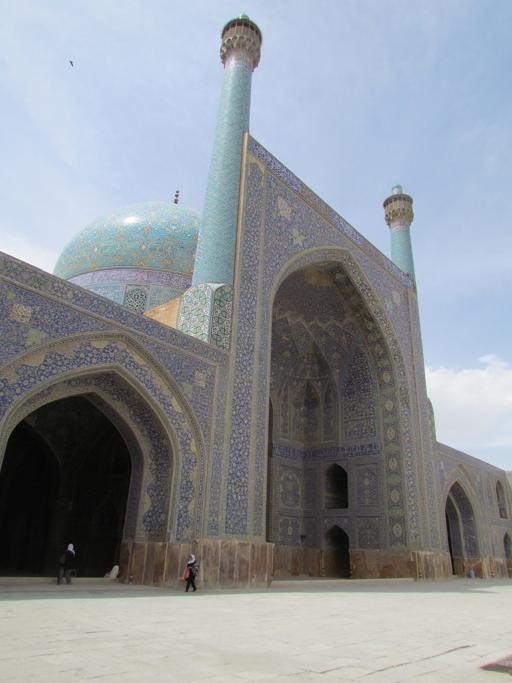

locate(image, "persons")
[[183, 552, 200, 595], [58, 543, 77, 585], [467, 566, 476, 587]]
